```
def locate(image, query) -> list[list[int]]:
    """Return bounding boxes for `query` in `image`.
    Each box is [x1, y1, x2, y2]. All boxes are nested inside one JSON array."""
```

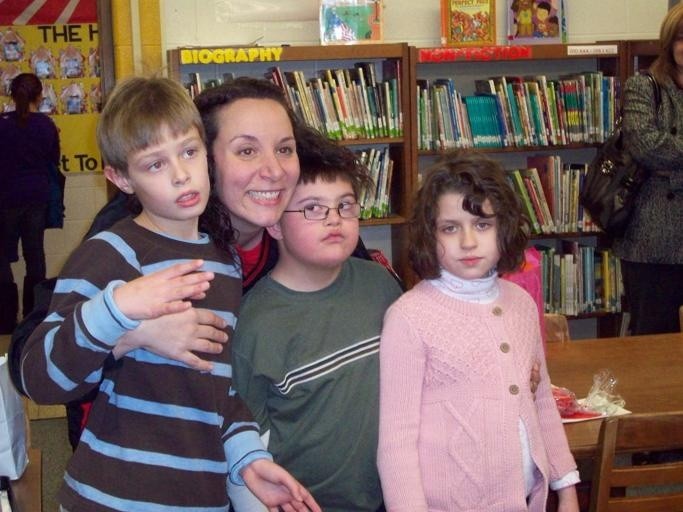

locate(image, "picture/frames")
[[506, 0, 569, 45], [440, 0, 497, 48]]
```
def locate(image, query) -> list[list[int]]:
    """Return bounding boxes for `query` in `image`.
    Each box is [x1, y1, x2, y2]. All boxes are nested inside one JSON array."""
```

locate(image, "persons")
[[622, 4, 682, 334], [1, 73, 64, 334], [376, 158, 583, 512], [11, 81, 540, 454], [233, 146, 400, 511], [18, 74, 327, 511]]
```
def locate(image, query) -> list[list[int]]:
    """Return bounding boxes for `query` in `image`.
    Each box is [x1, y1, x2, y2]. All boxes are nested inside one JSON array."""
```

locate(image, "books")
[[184, 62, 620, 315]]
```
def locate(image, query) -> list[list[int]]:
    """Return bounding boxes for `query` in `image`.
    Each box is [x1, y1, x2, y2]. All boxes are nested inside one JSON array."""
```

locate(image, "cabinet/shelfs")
[[409, 43, 631, 320], [597, 39, 659, 338], [168, 44, 414, 293]]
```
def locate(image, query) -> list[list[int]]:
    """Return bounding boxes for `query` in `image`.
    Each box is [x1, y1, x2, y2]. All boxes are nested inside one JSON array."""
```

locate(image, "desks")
[[544, 332, 683, 457]]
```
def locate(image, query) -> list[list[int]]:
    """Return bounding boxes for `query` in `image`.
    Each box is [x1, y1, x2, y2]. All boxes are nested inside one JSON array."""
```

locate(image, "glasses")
[[283, 202, 364, 221]]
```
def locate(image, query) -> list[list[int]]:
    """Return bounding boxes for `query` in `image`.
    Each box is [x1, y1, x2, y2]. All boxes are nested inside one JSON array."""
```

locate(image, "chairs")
[[546, 412, 683, 512]]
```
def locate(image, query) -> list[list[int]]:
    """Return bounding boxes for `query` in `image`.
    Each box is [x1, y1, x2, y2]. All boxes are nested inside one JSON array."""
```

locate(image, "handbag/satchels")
[[579, 135, 650, 237]]
